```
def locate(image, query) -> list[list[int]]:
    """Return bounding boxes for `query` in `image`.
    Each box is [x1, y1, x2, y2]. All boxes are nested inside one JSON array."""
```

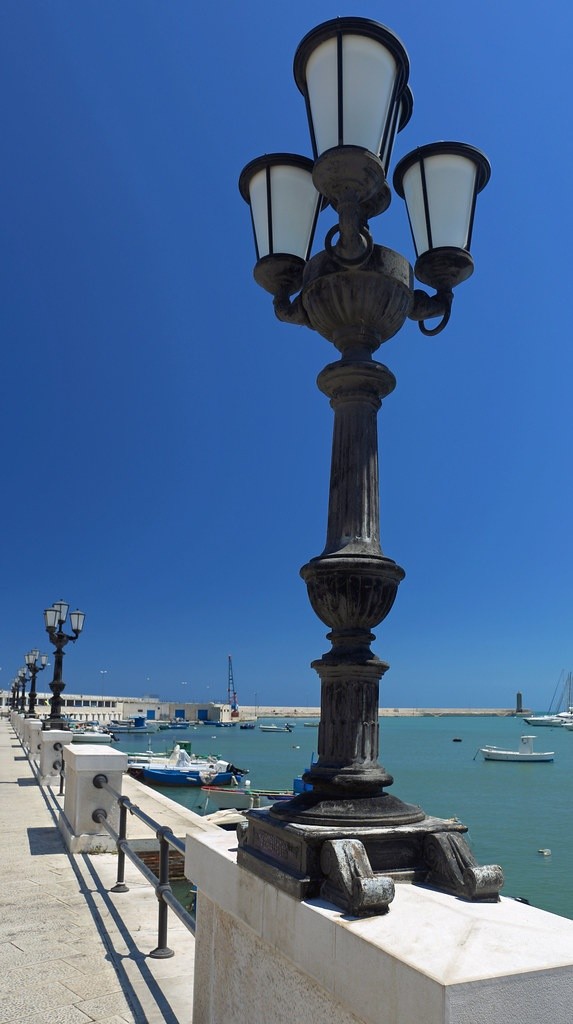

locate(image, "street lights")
[[225, 6, 509, 910], [10, 648, 47, 717], [37, 599, 83, 730]]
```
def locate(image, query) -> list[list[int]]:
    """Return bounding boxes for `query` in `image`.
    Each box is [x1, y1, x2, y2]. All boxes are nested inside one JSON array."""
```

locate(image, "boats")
[[62, 712, 319, 771], [523, 670, 572, 727], [129, 763, 249, 786], [200, 787, 294, 811], [480, 736, 555, 761]]
